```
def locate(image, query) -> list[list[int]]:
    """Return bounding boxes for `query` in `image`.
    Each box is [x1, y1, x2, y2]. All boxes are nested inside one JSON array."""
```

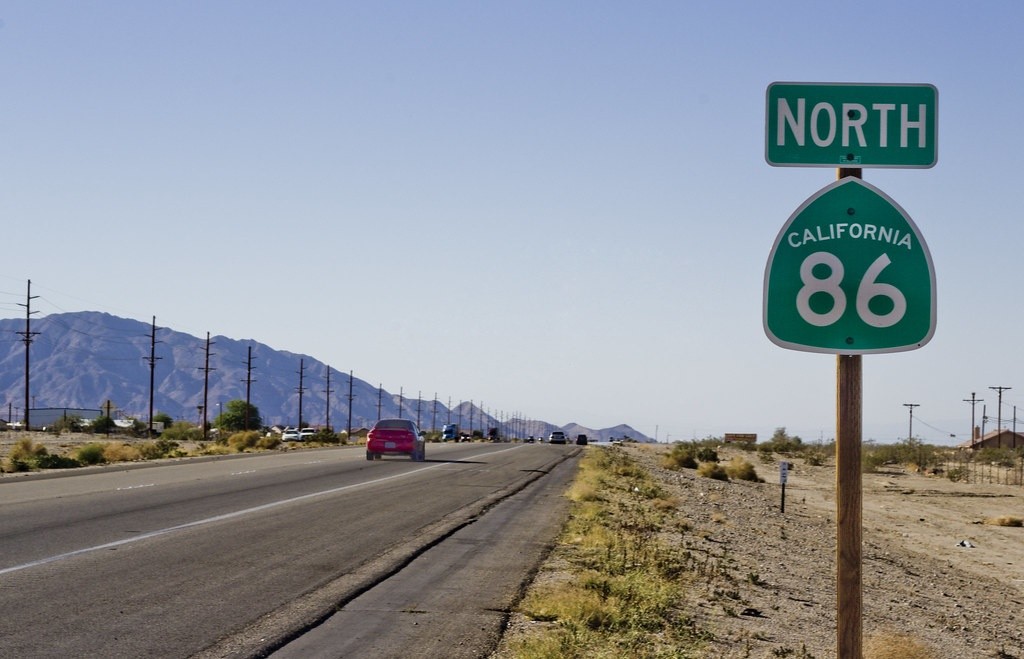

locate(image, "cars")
[[299, 427, 319, 441], [547, 431, 567, 444], [365, 416, 428, 461], [574, 434, 587, 445], [281, 429, 300, 441], [460, 433, 473, 442]]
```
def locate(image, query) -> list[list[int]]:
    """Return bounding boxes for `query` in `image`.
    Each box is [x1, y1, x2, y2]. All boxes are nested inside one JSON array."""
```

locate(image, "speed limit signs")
[[757, 175, 938, 357]]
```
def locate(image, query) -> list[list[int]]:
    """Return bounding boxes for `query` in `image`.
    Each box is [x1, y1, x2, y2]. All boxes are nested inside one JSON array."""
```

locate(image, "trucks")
[[441, 423, 461, 442]]
